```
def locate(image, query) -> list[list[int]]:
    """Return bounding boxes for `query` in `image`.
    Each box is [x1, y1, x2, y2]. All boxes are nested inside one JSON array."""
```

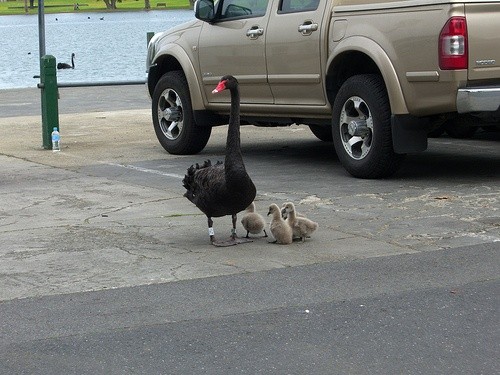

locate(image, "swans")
[[241, 202, 269, 238], [182, 75, 257, 247], [280, 202, 318, 243], [57, 53, 75, 69], [267, 204, 293, 244]]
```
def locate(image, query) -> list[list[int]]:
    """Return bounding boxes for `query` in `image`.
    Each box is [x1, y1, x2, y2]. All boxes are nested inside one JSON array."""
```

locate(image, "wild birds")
[[88, 17, 90, 19], [56, 18, 58, 20], [100, 17, 104, 20]]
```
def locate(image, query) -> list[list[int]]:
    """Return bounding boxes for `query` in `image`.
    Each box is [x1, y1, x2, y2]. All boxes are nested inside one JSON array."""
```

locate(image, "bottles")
[[51, 127, 60, 152]]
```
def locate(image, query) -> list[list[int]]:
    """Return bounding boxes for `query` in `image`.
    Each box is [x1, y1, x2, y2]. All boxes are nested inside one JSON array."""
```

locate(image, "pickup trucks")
[[143, 0, 499, 178]]
